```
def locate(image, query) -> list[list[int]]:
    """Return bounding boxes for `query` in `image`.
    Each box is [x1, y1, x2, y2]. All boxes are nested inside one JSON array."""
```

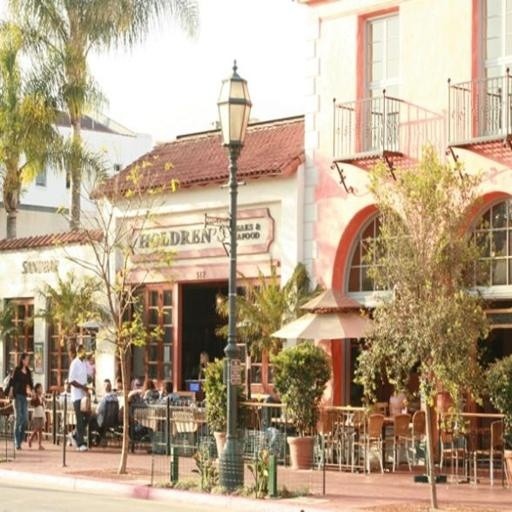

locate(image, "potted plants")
[[271, 340, 332, 470], [199, 357, 246, 458], [486, 353, 512, 489]]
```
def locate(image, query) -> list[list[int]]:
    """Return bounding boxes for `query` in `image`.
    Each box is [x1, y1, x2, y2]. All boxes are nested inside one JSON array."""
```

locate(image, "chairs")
[[318, 410, 504, 488]]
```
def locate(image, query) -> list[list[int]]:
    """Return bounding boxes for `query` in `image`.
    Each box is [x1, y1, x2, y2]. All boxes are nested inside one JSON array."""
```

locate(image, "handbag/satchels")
[[2, 374, 12, 394], [80, 395, 92, 416]]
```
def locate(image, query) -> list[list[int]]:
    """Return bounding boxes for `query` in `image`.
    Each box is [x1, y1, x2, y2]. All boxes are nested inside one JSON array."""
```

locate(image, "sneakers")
[[15, 436, 45, 450], [76, 444, 88, 452], [69, 433, 78, 447]]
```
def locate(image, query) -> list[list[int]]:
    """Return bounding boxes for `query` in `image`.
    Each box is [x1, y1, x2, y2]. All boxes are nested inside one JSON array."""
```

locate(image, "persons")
[[59, 376, 180, 446], [1, 353, 35, 451], [28, 383, 48, 451], [191, 350, 210, 382], [68, 343, 93, 452], [2, 366, 13, 392]]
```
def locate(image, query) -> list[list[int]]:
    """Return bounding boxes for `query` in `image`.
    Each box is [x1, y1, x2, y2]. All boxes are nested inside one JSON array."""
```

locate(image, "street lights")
[[209, 61, 255, 492]]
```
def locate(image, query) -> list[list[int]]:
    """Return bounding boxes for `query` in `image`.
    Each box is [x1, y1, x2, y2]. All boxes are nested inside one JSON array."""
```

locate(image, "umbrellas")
[[269, 287, 376, 340]]
